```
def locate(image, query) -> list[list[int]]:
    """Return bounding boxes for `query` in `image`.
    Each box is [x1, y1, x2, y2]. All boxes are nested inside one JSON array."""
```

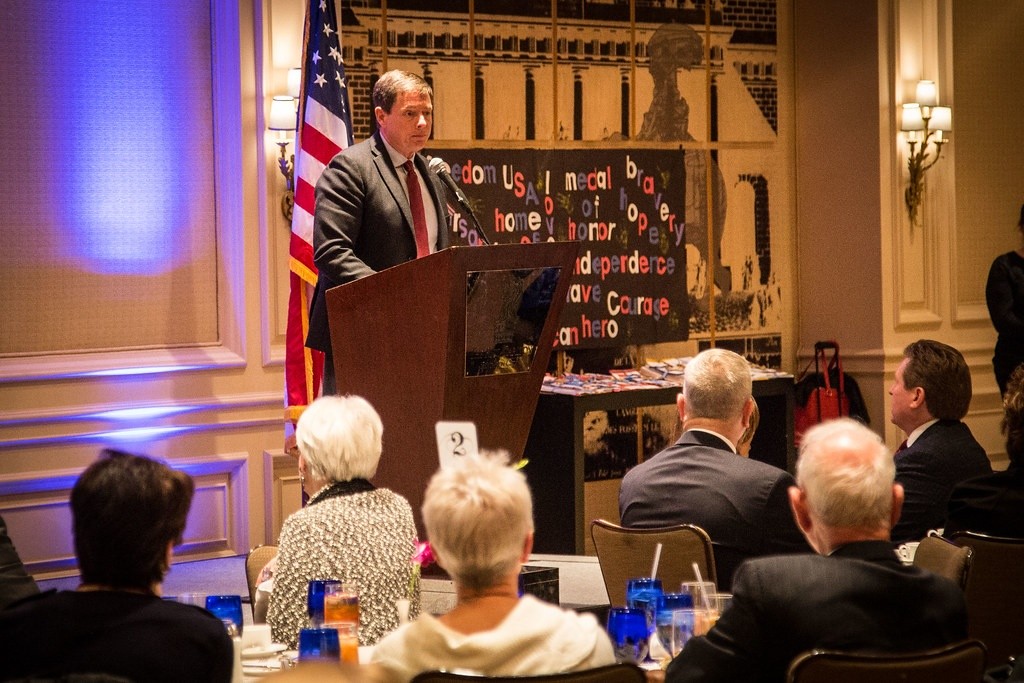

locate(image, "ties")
[[403, 160, 430, 258]]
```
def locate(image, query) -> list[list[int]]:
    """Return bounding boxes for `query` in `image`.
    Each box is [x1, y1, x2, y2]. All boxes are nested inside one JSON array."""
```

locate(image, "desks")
[[523, 363, 795, 557]]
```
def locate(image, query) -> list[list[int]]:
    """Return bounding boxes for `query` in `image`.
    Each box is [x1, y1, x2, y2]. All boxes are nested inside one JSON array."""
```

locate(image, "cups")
[[158, 580, 359, 671], [899, 528, 944, 562], [605, 577, 734, 667]]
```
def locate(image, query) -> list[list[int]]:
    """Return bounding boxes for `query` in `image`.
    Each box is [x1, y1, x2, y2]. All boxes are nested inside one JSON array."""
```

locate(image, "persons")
[[254, 392, 422, 651], [0, 448, 235, 683], [993, 363, 1024, 537], [888, 338, 997, 546], [985, 201, 1024, 399], [302, 67, 453, 396], [664, 416, 971, 683], [618, 347, 818, 594], [366, 455, 617, 683]]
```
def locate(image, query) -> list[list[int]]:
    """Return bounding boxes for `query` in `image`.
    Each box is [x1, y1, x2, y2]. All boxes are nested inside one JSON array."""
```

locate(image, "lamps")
[[900, 80, 952, 228], [267, 68, 302, 228]]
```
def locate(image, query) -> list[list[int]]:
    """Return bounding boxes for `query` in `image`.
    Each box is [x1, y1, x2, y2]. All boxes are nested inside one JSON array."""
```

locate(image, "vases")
[[396, 599, 411, 627]]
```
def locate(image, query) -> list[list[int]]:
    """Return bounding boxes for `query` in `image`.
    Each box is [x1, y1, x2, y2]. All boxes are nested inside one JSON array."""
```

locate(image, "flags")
[[281, 0, 358, 458]]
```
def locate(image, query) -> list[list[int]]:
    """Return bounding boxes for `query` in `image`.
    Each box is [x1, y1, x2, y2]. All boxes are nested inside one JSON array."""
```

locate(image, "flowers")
[[408, 539, 436, 599]]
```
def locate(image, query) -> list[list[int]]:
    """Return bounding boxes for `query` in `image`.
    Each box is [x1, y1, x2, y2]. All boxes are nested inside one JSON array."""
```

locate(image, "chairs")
[[245, 520, 1024, 683]]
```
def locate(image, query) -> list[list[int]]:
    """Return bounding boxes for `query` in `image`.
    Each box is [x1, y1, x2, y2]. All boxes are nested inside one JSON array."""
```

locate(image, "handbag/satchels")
[[794, 341, 848, 447], [795, 369, 871, 425]]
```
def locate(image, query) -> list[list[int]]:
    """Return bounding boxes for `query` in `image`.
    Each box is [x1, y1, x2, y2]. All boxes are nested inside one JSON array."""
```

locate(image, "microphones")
[[429, 158, 473, 213]]
